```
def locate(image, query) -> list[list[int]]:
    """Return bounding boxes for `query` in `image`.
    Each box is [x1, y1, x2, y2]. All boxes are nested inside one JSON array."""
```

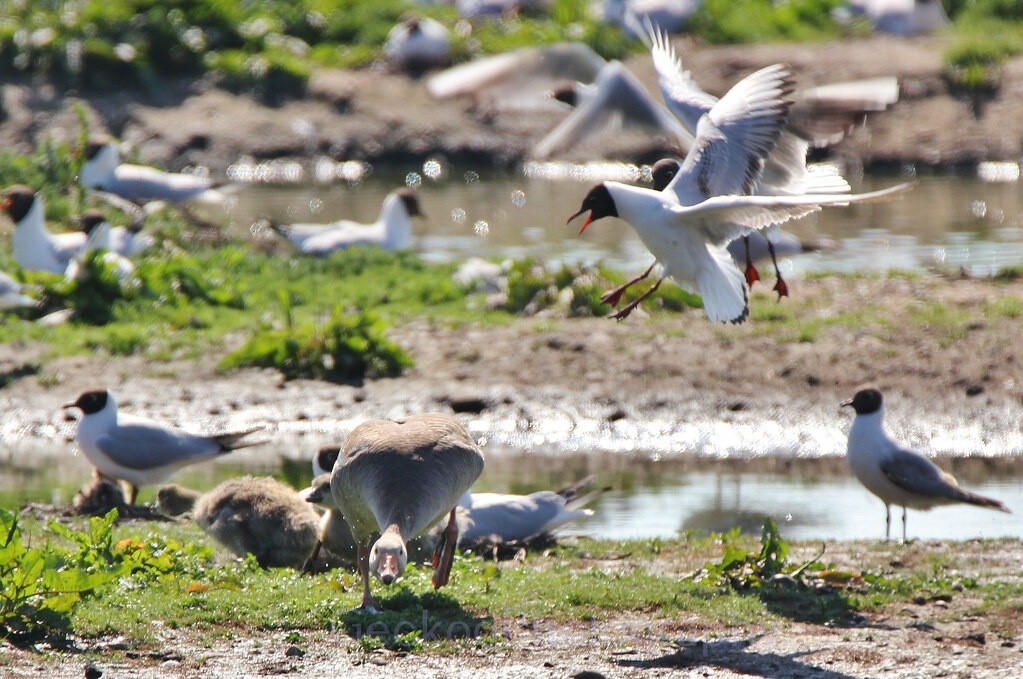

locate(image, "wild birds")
[[0, 184, 157, 271], [331, 414, 485, 618], [79, 133, 232, 206], [386, 14, 827, 266], [841, 388, 1011, 543], [645, 18, 851, 304], [66, 209, 142, 288], [265, 188, 426, 259], [566, 64, 911, 325], [72, 445, 613, 578], [0, 271, 45, 310], [63, 388, 272, 507]]
